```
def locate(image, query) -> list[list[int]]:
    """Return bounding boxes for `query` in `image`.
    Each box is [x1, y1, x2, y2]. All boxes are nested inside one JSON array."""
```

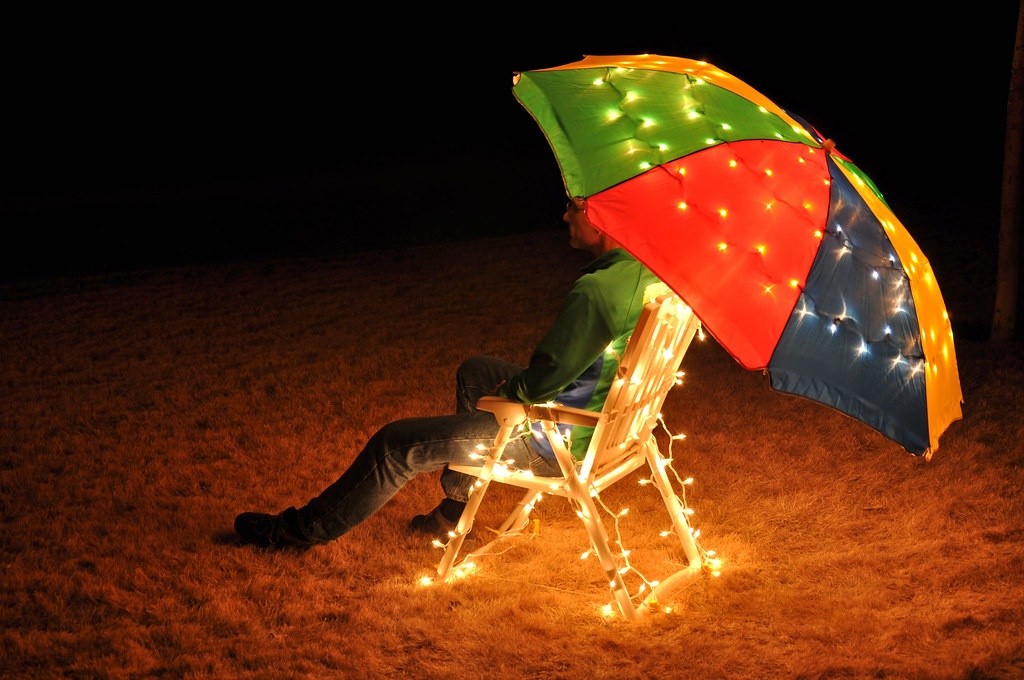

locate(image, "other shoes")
[[410, 506, 475, 540], [234, 506, 314, 556]]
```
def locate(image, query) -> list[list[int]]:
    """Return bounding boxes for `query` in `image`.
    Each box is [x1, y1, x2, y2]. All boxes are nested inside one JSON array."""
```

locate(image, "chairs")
[[438, 294, 704, 621]]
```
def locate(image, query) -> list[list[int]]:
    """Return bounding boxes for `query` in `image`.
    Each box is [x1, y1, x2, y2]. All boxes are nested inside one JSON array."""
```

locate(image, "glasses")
[[566, 202, 584, 216]]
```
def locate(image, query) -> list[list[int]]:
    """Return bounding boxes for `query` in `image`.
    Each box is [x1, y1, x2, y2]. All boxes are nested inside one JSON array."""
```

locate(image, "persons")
[[233, 198, 664, 555]]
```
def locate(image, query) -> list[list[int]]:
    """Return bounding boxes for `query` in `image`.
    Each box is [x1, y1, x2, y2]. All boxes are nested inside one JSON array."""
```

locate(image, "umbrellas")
[[512, 53, 966, 463]]
